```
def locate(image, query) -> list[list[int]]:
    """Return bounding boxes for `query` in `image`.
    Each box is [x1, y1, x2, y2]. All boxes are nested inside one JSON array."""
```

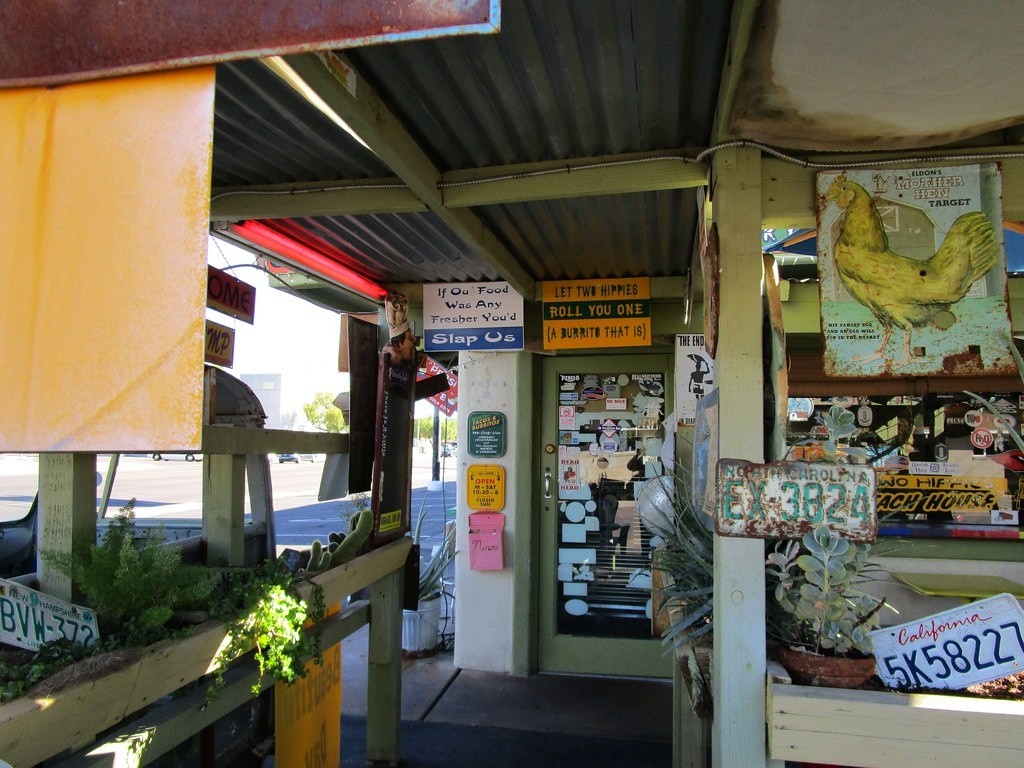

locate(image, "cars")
[[441, 443, 452, 457], [278, 453, 319, 464], [146, 454, 203, 462]]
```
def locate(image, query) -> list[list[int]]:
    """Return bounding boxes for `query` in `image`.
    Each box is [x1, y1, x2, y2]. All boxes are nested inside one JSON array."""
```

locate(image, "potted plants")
[[402, 491, 462, 660], [766, 524, 883, 689]]
